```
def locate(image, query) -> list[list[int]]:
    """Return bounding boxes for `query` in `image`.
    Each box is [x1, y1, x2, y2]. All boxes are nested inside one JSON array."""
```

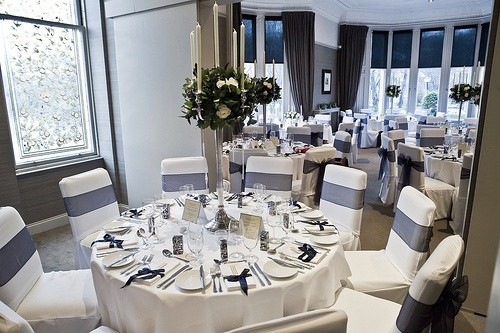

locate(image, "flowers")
[[181, 68, 254, 130], [448, 83, 484, 104], [251, 75, 282, 106], [385, 85, 400, 98], [285, 110, 300, 118]]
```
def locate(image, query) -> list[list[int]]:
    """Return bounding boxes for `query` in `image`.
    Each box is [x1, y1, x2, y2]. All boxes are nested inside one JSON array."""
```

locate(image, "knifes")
[[200, 265, 206, 295], [248, 262, 270, 286], [157, 266, 192, 290]]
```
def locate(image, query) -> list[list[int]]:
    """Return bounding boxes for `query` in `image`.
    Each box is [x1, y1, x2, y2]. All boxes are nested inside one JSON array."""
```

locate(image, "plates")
[[102, 251, 134, 267], [266, 195, 283, 204], [265, 260, 299, 277], [175, 269, 212, 290], [102, 221, 130, 234], [312, 235, 339, 245], [299, 210, 324, 219]]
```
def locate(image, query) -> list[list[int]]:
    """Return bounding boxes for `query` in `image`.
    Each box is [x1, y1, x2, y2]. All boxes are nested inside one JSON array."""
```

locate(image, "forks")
[[209, 264, 222, 293], [120, 254, 154, 275]]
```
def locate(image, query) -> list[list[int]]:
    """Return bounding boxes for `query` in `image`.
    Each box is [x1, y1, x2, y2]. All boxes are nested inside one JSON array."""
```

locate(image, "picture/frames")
[[322, 69, 332, 94]]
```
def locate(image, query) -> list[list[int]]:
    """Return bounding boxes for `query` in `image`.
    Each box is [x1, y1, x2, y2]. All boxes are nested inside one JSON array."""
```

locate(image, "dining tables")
[[91, 192, 352, 332], [222, 137, 309, 190], [421, 144, 475, 169]]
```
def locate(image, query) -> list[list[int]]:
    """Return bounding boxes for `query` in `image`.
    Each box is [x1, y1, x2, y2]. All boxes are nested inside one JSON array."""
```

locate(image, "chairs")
[[0, 109, 479, 333]]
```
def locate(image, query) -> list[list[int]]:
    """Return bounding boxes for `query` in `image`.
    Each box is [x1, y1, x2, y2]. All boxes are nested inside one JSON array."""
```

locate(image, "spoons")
[[137, 228, 149, 246], [163, 249, 190, 264]]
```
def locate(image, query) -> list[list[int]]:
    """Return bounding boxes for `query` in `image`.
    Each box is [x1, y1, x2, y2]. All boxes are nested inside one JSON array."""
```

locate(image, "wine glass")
[[140, 182, 297, 265], [239, 130, 294, 152]]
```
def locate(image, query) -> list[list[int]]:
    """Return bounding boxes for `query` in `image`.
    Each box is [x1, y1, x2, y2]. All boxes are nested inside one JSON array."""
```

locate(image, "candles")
[[196, 22, 202, 92], [453, 61, 480, 95], [240, 23, 245, 90], [213, 1, 220, 67], [189, 30, 194, 78], [233, 29, 237, 73], [254, 60, 256, 76], [272, 59, 275, 82]]
